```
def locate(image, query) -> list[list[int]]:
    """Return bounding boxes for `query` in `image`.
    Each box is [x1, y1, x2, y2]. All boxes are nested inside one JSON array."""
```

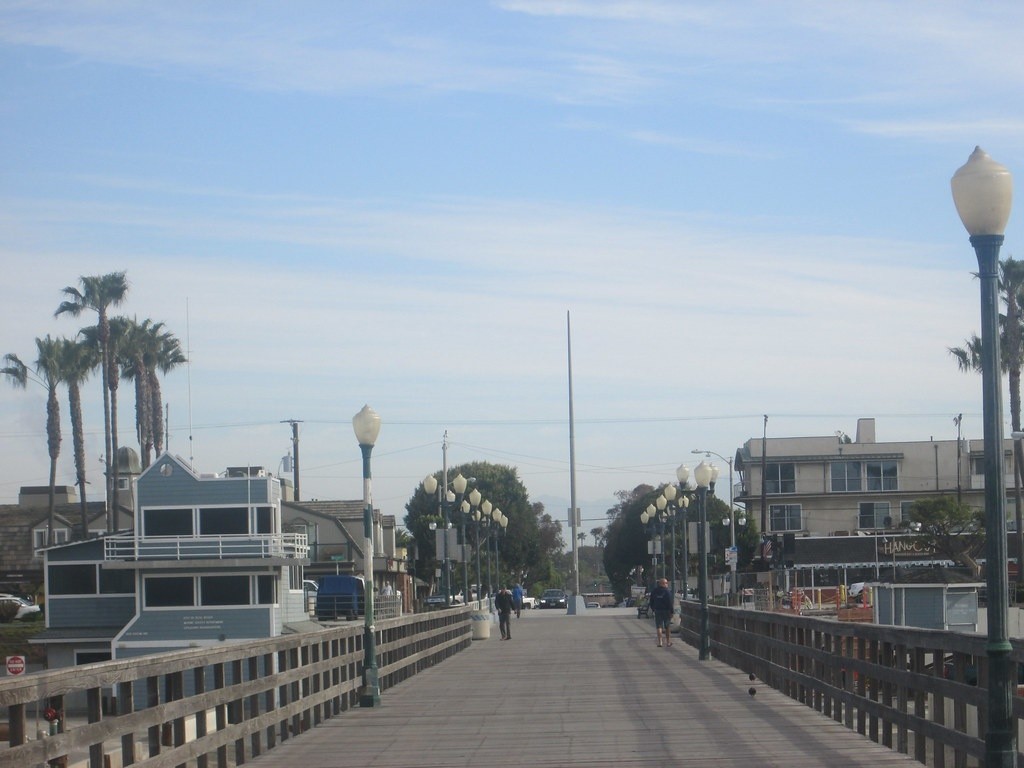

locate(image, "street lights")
[[351, 403, 384, 708], [691, 448, 736, 593], [953, 414, 963, 503], [423, 473, 508, 608], [640, 459, 720, 662], [949, 144, 1024, 768]]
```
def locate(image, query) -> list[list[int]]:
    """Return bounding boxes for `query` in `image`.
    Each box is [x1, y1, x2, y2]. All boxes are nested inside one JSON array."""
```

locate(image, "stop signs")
[[6, 656, 25, 676]]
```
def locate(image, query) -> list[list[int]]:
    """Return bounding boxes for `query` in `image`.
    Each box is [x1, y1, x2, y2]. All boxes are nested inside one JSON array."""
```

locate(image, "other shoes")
[[657, 644, 662, 647], [504, 637, 511, 640], [500, 637, 505, 640], [667, 642, 672, 647]]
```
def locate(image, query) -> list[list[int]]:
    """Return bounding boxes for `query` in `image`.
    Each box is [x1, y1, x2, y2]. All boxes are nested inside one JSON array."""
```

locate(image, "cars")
[[427, 583, 488, 608], [850, 581, 868, 604], [0, 593, 42, 619], [499, 590, 536, 610], [602, 604, 616, 608], [303, 579, 319, 614], [539, 589, 569, 609]]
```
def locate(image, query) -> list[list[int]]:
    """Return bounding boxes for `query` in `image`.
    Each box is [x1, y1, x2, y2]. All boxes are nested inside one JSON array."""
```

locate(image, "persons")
[[513, 581, 524, 618], [381, 580, 394, 596], [467, 584, 473, 602], [650, 578, 674, 647], [495, 584, 517, 641]]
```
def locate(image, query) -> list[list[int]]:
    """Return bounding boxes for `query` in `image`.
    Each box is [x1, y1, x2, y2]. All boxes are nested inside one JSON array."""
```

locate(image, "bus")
[[587, 601, 600, 608]]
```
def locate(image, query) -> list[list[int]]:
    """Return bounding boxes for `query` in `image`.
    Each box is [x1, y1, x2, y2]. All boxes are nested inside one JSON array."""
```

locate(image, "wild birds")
[[749, 686, 756, 697], [749, 671, 756, 683]]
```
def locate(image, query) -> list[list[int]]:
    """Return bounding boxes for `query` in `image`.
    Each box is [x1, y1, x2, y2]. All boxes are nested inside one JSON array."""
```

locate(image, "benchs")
[[638, 603, 651, 619]]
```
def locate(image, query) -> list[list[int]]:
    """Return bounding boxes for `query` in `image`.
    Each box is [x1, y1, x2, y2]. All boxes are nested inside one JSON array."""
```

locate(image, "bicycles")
[[778, 583, 814, 610]]
